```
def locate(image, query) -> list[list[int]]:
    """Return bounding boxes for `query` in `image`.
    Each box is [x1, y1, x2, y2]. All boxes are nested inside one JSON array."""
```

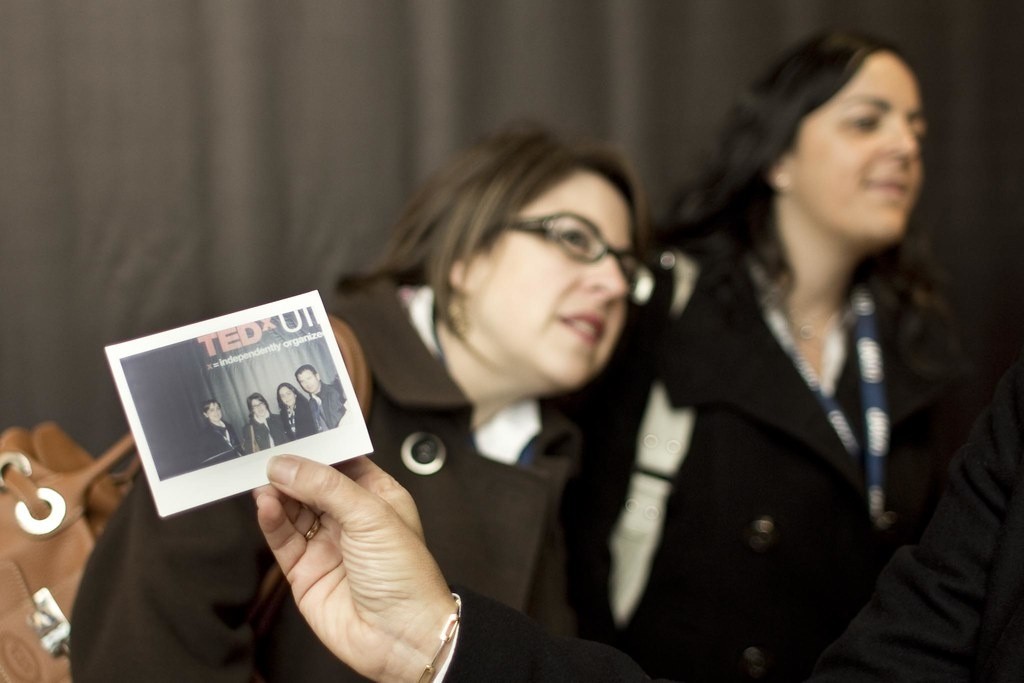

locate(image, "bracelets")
[[418, 598, 461, 683]]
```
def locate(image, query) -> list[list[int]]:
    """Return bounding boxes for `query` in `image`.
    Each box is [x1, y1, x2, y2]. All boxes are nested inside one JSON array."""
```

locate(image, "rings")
[[304, 515, 320, 540]]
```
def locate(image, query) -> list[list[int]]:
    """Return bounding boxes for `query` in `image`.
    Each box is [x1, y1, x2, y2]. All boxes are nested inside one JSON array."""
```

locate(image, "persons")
[[557, 24, 997, 683], [201, 400, 242, 458], [242, 393, 289, 451], [295, 364, 345, 432], [254, 359, 1024, 683], [72, 133, 658, 683], [277, 382, 316, 441]]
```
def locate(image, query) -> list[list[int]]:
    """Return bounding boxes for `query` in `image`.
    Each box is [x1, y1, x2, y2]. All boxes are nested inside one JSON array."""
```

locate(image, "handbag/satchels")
[[0, 422, 119, 683]]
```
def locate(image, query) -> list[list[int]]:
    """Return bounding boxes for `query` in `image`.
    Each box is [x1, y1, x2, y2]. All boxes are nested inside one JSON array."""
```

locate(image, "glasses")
[[252, 402, 265, 409], [502, 212, 657, 305]]
